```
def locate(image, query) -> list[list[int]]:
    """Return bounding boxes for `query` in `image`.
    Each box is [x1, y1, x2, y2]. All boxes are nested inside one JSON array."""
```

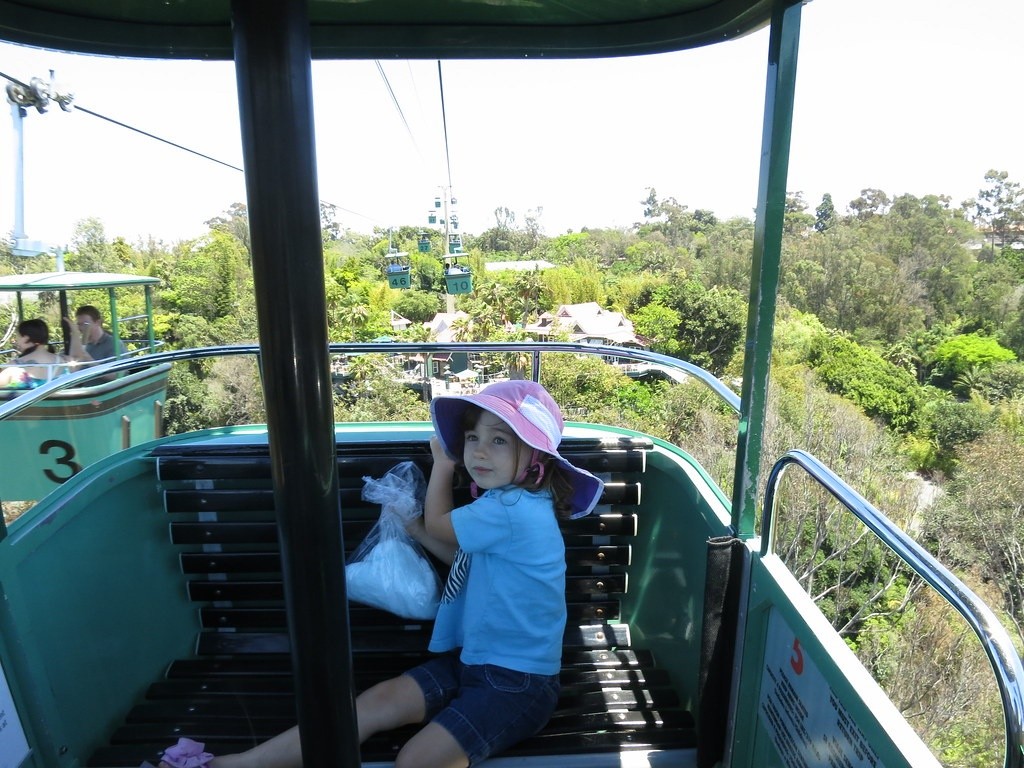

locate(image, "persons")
[[64, 306, 131, 386], [444, 263, 451, 275], [1, 316, 71, 394], [388, 261, 404, 272], [151, 379, 572, 767]]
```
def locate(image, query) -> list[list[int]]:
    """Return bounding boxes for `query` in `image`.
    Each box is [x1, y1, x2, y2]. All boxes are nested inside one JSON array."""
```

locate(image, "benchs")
[[84, 436, 678, 766]]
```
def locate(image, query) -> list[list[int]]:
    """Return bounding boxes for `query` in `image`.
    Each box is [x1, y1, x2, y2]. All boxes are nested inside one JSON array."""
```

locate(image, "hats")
[[430, 381, 604, 520]]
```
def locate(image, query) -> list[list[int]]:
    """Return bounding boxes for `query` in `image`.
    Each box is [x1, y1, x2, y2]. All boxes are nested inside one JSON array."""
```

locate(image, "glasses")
[[77, 321, 91, 330]]
